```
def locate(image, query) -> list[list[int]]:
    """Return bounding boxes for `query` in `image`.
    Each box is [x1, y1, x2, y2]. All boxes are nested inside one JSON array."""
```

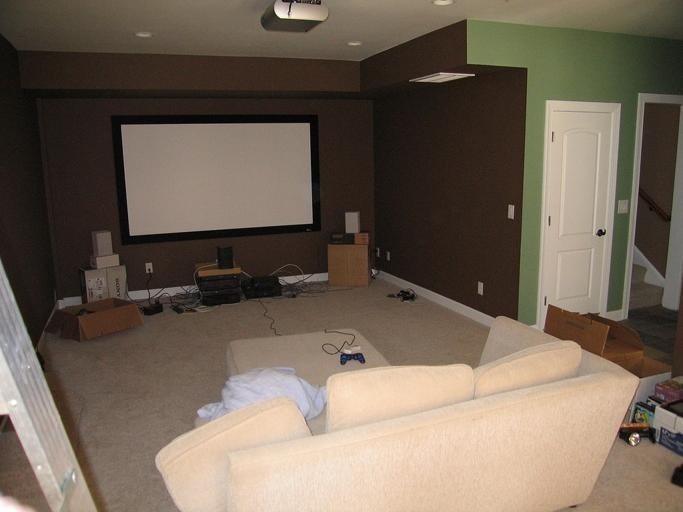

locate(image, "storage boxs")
[[541, 302, 646, 365], [42, 297, 144, 344], [77, 230, 128, 305], [613, 350, 675, 428], [653, 396, 682, 456], [328, 210, 371, 245]]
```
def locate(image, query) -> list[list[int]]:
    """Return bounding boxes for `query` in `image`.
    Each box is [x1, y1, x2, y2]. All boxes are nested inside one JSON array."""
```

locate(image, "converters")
[[144, 303, 163, 315]]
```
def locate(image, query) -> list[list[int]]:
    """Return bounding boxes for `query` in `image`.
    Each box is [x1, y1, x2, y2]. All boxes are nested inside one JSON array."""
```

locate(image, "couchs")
[[222, 327, 394, 399], [145, 314, 642, 510]]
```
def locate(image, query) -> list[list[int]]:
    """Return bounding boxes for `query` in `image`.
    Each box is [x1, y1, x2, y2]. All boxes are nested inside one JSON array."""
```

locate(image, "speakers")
[[92, 230, 113, 256], [345, 211, 361, 233]]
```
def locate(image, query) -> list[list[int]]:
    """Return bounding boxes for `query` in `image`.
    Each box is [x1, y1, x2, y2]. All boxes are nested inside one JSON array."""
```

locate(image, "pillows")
[[321, 362, 475, 437], [471, 339, 582, 404], [152, 394, 312, 472]]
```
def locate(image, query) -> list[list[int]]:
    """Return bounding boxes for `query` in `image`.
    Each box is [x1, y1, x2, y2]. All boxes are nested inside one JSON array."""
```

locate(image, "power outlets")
[[144, 262, 154, 274]]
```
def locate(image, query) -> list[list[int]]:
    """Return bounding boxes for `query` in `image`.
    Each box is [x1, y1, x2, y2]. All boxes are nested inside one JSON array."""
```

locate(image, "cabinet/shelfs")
[[325, 241, 373, 287]]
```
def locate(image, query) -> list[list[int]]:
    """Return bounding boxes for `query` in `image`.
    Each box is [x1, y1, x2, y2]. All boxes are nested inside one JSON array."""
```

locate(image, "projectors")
[[261, 0, 328, 32]]
